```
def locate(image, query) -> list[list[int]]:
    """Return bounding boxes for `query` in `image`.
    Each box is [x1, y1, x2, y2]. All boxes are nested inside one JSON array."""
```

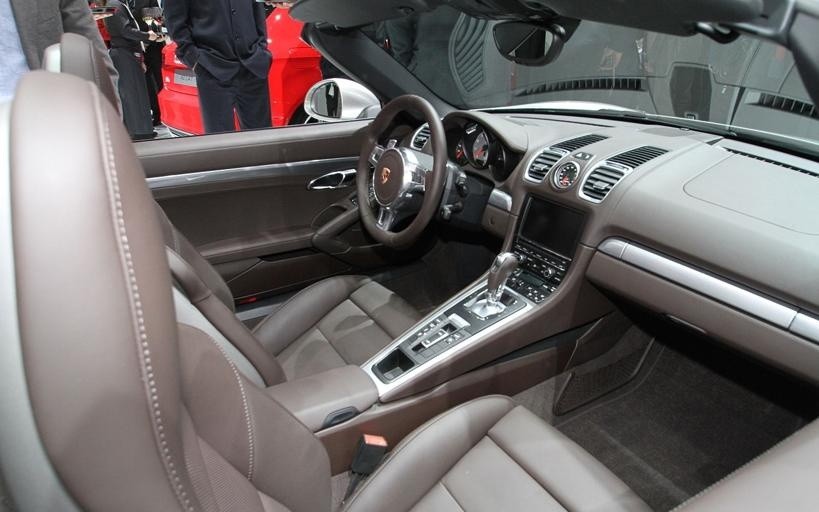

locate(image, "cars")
[[157, 6, 322, 135]]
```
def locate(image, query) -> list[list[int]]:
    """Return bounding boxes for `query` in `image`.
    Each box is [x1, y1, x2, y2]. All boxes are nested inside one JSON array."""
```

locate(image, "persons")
[[571, 22, 643, 89], [0, 1, 124, 127], [264, 1, 416, 103], [88, 1, 167, 142], [159, 1, 274, 137]]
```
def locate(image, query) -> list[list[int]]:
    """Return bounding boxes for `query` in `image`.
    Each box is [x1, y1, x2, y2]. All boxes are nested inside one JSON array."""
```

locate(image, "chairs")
[[1, 30, 654, 512]]
